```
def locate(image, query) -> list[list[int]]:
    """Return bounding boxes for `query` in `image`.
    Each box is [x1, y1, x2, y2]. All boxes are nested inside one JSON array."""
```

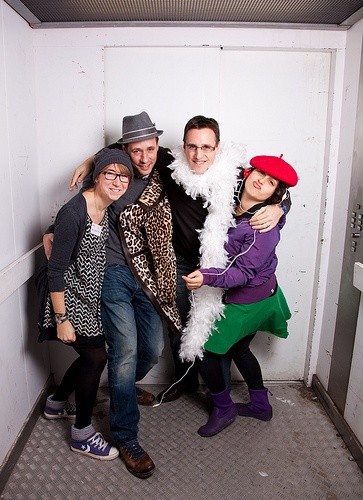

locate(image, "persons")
[[43, 112, 185, 479], [181, 154, 299, 437], [34, 147, 134, 462], [68, 115, 292, 404]]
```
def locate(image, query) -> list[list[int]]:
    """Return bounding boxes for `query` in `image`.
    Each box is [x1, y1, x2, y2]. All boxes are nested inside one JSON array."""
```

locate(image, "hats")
[[251, 153, 299, 189], [116, 112, 164, 144], [93, 148, 134, 181]]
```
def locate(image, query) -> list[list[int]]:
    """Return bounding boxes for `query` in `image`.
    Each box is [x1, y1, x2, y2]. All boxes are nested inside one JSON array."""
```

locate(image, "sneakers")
[[136, 385, 155, 405], [43, 394, 77, 419], [70, 424, 120, 461], [119, 441, 156, 479]]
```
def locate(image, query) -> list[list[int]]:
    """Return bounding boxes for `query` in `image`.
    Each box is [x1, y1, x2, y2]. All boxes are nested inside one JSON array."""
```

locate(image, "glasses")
[[101, 169, 131, 184], [185, 140, 220, 152]]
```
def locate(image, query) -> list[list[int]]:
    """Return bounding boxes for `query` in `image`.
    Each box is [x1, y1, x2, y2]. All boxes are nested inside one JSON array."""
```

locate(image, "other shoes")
[[157, 379, 199, 404]]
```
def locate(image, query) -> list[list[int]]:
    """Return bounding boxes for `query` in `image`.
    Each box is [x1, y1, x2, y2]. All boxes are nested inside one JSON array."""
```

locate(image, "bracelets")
[[54, 312, 69, 325]]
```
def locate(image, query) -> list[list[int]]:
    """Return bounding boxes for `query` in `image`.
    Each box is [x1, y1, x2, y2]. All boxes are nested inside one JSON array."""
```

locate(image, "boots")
[[197, 382, 239, 437], [234, 386, 273, 421]]
